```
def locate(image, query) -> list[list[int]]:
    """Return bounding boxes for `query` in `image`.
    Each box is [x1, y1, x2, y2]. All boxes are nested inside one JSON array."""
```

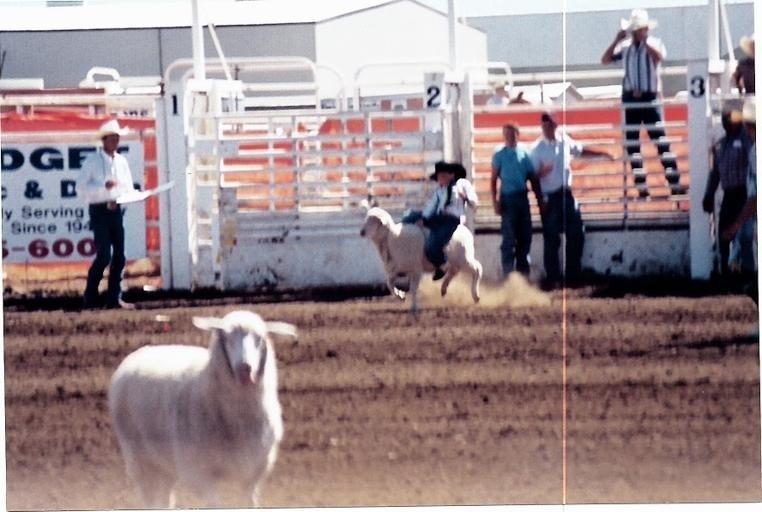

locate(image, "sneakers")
[[432, 262, 450, 281], [83, 288, 134, 309]]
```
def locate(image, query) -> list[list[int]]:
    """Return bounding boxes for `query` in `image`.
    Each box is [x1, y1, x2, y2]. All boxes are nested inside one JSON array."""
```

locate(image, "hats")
[[430, 161, 466, 181], [620, 10, 657, 32], [740, 35, 756, 59], [96, 120, 129, 139], [731, 95, 756, 125]]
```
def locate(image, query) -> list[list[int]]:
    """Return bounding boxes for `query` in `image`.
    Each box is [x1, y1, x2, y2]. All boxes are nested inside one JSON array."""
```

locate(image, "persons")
[[601, 9, 688, 199], [529, 111, 616, 283], [401, 160, 478, 280], [75, 119, 135, 311], [703, 30, 758, 290], [491, 122, 543, 282]]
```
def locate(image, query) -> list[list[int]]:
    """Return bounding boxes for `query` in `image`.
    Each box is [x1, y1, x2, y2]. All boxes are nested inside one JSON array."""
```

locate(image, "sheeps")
[[359, 204, 483, 313], [106, 310, 298, 509]]
